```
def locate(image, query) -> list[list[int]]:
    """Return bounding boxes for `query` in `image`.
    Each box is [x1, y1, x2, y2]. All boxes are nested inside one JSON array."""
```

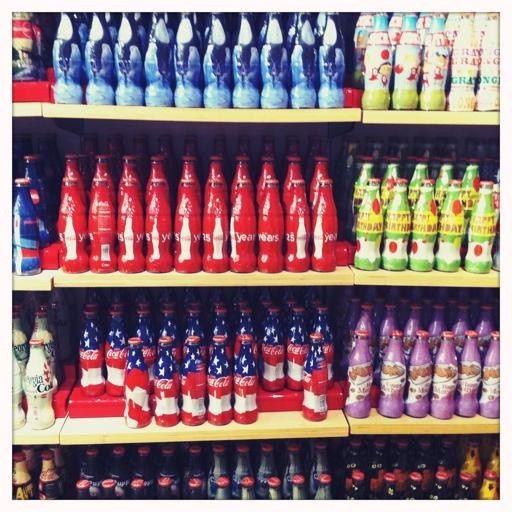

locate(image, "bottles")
[[13, 292, 500, 431], [12, 135, 499, 277], [11, 435, 501, 499], [13, 13, 501, 112]]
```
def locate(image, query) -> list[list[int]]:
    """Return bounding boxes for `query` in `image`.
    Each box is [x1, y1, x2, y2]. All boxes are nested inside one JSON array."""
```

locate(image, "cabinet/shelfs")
[[12, 103, 500, 445]]
